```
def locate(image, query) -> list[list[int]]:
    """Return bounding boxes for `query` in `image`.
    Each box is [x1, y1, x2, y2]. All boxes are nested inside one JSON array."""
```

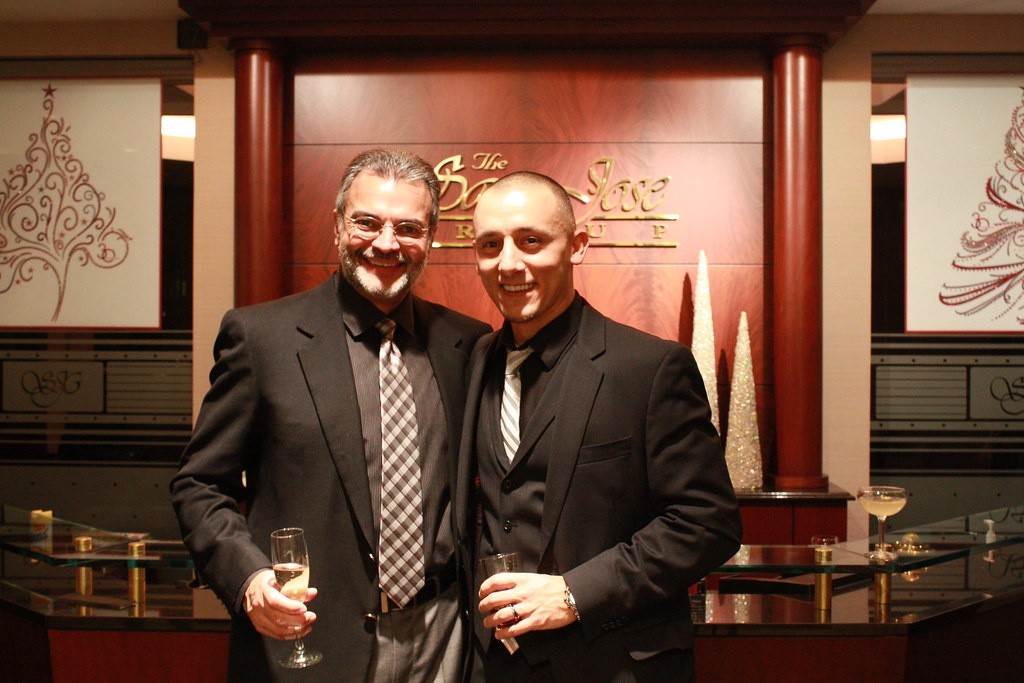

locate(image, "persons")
[[456, 170, 743, 683], [170, 147, 495, 683]]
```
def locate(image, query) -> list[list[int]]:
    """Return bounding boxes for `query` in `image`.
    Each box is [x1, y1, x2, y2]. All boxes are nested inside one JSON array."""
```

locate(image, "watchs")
[[564, 583, 581, 623]]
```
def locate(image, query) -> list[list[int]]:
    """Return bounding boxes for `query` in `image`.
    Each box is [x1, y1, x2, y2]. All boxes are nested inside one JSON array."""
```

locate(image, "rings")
[[509, 603, 520, 621]]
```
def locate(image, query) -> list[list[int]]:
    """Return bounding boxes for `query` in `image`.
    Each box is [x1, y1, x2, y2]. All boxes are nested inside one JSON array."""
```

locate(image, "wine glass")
[[856, 486, 908, 560], [268, 527, 330, 668]]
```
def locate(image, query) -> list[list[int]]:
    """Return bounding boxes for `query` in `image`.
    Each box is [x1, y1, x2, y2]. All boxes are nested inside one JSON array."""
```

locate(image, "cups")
[[480, 552, 527, 627]]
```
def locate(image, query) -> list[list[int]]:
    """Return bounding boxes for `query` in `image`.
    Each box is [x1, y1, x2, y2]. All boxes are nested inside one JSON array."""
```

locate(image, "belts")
[[378, 567, 452, 614]]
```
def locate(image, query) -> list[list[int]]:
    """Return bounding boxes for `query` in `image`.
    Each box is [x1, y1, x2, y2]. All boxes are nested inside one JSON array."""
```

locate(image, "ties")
[[499, 346, 534, 465], [375, 318, 426, 610]]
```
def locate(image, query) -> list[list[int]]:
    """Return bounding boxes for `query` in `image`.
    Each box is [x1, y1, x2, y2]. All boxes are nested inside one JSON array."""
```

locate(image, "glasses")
[[343, 213, 432, 246]]
